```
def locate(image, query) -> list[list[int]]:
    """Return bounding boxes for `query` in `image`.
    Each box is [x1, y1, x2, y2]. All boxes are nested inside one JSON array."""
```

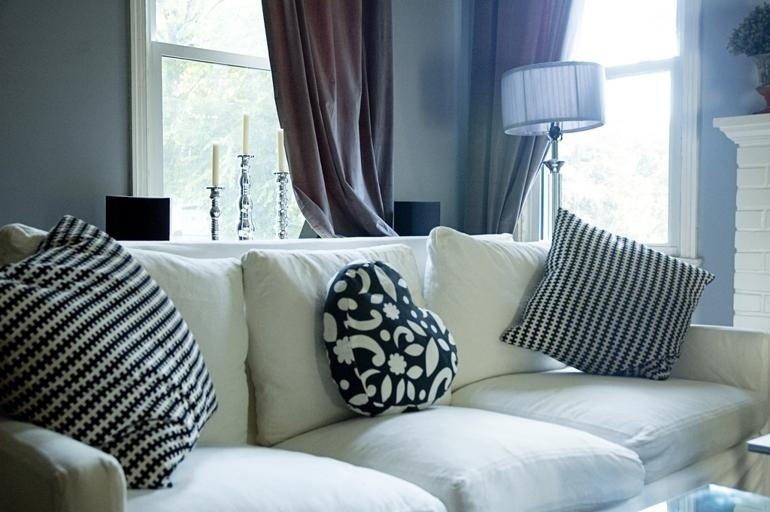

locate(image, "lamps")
[[500, 59, 606, 238]]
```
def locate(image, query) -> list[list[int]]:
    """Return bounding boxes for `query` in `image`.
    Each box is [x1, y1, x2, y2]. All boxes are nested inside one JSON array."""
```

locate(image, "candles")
[[243, 113, 249, 156], [212, 144, 219, 185], [277, 128, 285, 172]]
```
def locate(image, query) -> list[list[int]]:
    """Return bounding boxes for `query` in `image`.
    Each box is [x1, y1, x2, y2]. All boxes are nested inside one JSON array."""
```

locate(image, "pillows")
[[1, 212, 219, 491], [321, 258, 461, 418], [499, 207, 717, 380]]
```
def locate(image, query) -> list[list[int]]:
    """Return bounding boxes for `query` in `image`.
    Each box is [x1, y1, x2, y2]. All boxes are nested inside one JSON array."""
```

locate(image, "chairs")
[[103, 194, 171, 241]]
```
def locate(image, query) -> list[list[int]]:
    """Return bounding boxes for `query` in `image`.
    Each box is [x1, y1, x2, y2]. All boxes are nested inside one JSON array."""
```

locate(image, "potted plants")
[[725, 2, 770, 116]]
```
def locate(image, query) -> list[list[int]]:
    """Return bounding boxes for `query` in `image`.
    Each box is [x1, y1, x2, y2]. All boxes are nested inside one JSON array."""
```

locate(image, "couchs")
[[0, 222, 770, 511]]
